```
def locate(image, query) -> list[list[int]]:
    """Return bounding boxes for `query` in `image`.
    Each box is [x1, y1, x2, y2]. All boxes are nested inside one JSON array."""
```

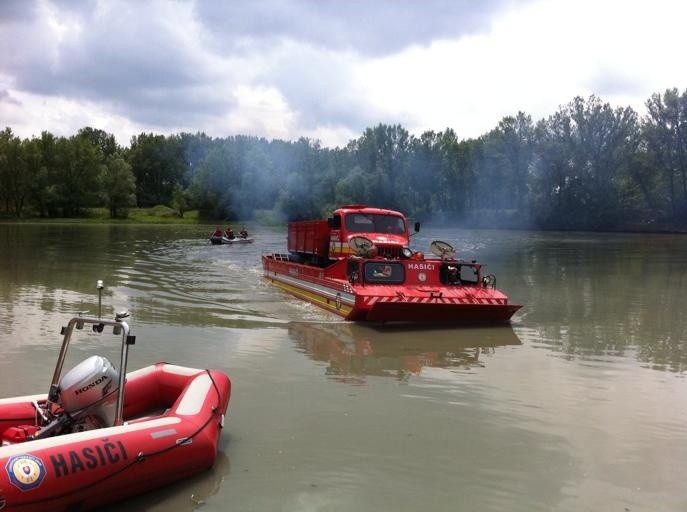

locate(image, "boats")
[[261, 241, 523, 324], [209, 234, 255, 245], [0, 279, 232, 512]]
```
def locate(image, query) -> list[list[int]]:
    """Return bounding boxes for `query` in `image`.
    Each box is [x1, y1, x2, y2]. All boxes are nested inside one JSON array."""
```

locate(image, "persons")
[[213, 224, 222, 237], [239, 226, 248, 239], [223, 223, 235, 239]]
